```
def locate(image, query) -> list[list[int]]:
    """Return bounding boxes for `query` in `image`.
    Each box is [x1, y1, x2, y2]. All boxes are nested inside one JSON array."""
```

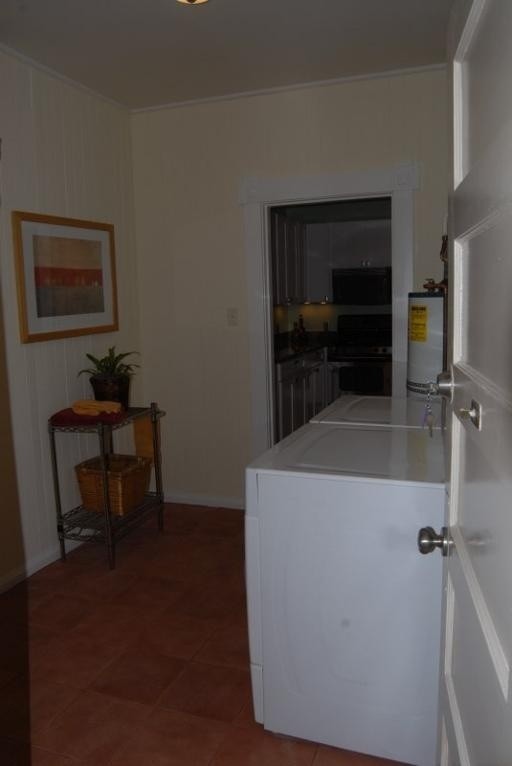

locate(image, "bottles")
[[299, 319, 309, 348], [292, 321, 305, 352]]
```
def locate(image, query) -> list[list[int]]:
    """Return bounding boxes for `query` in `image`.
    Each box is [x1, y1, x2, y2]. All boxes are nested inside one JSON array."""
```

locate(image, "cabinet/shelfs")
[[332, 218, 391, 268], [276, 346, 327, 443], [303, 223, 332, 302], [272, 212, 304, 306], [49, 403, 166, 568]]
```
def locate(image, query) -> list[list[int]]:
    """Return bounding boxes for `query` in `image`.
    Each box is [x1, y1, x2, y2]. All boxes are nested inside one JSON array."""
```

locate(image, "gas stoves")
[[328, 314, 392, 355]]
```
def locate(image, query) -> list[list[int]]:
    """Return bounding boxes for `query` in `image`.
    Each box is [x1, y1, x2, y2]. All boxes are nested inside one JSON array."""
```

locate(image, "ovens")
[[328, 355, 392, 401]]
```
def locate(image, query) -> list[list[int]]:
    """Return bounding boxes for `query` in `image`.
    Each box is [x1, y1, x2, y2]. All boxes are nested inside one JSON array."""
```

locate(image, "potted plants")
[[77, 346, 141, 411]]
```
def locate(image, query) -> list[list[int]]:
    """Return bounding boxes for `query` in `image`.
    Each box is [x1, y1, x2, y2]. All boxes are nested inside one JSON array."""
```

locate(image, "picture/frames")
[[11, 210, 119, 344]]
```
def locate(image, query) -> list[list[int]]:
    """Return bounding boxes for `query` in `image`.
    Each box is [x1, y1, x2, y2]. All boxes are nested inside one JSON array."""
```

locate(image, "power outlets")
[[227, 308, 239, 326]]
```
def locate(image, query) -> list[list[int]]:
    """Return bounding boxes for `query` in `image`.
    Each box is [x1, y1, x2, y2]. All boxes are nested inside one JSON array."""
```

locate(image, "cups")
[[304, 297, 311, 304], [320, 295, 328, 304], [360, 257, 371, 267]]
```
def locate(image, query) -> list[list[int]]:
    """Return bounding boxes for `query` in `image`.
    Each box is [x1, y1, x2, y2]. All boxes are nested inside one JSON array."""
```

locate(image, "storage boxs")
[[74, 454, 152, 516]]
